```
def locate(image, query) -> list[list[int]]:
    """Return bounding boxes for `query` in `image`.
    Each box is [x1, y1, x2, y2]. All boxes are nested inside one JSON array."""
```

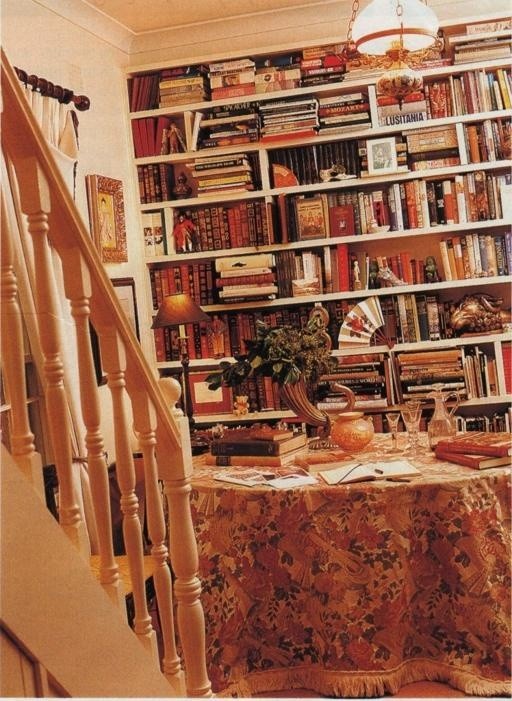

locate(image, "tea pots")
[[425, 382, 462, 450]]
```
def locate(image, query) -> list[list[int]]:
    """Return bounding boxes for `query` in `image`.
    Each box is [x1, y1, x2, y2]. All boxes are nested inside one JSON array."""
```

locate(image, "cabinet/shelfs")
[[120, 10, 511, 427]]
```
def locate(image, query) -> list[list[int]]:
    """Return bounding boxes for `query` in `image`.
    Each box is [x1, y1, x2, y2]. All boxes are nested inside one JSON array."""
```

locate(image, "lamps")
[[148, 292, 216, 453], [335, 0, 445, 114]]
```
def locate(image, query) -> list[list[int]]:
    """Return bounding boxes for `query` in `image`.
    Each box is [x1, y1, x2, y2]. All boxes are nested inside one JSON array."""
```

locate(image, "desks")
[[141, 419, 511, 698]]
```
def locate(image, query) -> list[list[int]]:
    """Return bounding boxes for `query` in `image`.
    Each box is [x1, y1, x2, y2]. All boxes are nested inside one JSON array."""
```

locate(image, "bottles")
[[330, 411, 374, 455]]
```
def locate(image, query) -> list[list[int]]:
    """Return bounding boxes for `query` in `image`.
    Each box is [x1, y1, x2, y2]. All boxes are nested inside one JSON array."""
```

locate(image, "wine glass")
[[401, 400, 424, 457], [385, 413, 401, 455]]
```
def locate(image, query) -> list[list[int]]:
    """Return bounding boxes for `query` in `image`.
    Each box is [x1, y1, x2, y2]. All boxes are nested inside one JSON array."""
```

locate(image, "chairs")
[[41, 460, 180, 647]]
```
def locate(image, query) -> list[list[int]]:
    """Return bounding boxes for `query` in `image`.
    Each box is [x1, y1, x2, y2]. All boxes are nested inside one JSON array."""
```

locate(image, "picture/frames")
[[88, 275, 145, 387], [365, 134, 399, 177], [84, 172, 129, 269], [294, 197, 328, 242]]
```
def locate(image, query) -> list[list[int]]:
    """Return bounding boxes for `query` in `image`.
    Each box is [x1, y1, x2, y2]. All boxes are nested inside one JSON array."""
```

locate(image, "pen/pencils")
[[374, 468, 383, 475], [386, 477, 410, 482]]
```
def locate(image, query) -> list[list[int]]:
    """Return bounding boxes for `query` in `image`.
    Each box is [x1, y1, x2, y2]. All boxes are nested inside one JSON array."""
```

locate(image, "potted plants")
[[201, 313, 358, 454]]
